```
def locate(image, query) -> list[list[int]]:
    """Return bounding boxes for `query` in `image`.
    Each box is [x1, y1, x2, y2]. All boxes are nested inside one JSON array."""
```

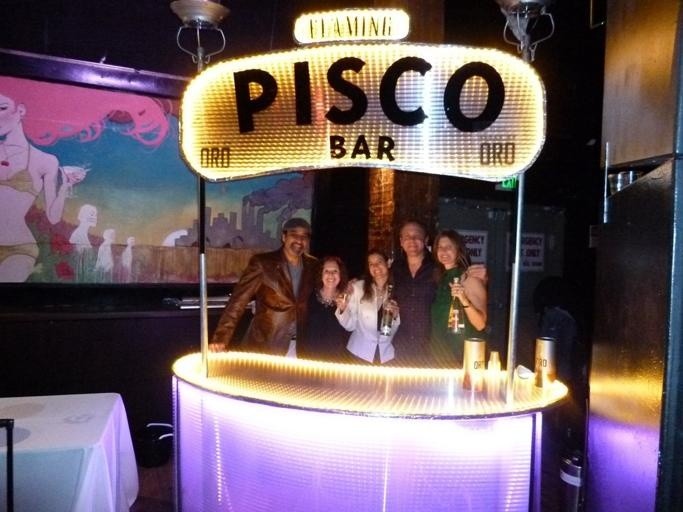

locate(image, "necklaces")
[[316, 291, 340, 309]]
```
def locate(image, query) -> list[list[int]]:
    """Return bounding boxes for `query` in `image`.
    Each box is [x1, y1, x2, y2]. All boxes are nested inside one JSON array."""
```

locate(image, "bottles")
[[486, 351, 500, 399], [380, 284, 395, 336], [446, 277, 466, 337]]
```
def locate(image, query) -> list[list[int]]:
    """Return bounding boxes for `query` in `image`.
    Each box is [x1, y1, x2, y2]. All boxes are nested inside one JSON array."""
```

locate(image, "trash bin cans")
[[558, 455, 586, 511]]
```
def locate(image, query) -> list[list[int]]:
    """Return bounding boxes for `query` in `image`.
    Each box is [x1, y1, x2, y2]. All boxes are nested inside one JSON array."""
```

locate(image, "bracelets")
[[461, 304, 472, 309]]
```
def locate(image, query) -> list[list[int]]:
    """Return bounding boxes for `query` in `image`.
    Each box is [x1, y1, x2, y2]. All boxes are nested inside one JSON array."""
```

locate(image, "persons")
[[208, 216, 317, 359], [309, 255, 349, 358], [428, 230, 488, 369], [388, 218, 487, 368], [334, 248, 401, 368]]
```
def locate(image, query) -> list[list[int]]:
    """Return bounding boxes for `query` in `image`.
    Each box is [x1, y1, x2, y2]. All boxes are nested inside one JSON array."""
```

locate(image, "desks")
[[0, 391, 124, 511]]
